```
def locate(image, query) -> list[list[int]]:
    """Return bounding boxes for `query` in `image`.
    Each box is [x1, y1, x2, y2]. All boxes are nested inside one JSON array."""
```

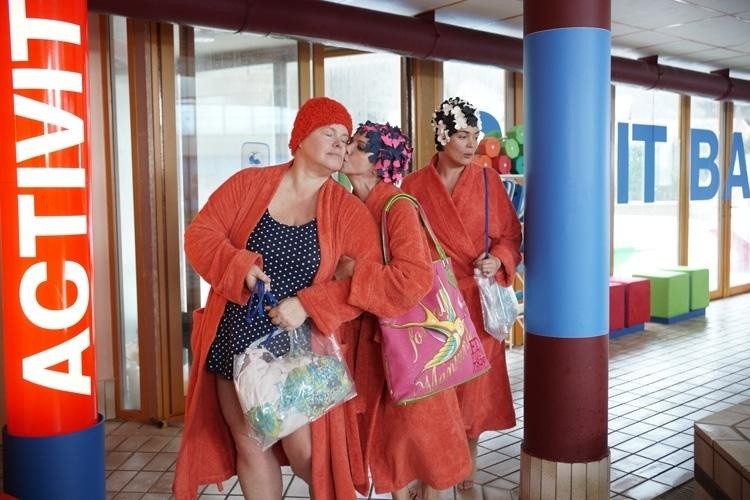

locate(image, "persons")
[[402, 97, 522, 500], [172, 95, 384, 500], [335, 120, 477, 500]]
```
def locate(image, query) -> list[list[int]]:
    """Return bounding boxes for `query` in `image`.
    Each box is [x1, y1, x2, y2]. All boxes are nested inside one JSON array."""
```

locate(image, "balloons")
[[479, 126, 524, 174]]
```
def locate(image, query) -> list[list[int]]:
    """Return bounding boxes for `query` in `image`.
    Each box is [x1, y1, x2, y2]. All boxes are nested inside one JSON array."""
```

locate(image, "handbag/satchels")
[[232, 276, 359, 453], [376, 193, 492, 406], [472, 250, 520, 342]]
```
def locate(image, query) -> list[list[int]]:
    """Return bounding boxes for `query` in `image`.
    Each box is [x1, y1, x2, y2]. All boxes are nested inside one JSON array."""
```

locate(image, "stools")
[[608, 265, 711, 344]]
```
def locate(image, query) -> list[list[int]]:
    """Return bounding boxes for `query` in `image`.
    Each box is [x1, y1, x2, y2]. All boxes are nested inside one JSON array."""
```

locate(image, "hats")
[[356, 120, 414, 184], [430, 96, 482, 152], [289, 96, 353, 154]]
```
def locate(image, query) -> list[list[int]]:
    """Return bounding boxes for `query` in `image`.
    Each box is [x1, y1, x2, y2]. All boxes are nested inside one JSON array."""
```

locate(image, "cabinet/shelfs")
[[493, 172, 525, 352]]
